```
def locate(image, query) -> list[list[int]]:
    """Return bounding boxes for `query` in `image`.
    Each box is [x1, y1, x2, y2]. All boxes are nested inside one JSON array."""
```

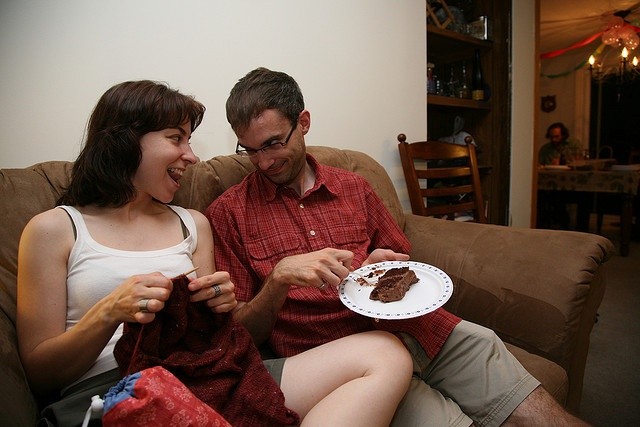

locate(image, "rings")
[[212, 285, 221, 296], [320, 283, 326, 289], [139, 299, 149, 313]]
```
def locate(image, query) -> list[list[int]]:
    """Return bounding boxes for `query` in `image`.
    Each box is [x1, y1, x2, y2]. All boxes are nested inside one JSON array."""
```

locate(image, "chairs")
[[398, 134, 487, 225]]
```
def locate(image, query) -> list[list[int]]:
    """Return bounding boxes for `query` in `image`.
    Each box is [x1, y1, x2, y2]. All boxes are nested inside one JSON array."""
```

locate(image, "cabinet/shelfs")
[[426, 0, 512, 227]]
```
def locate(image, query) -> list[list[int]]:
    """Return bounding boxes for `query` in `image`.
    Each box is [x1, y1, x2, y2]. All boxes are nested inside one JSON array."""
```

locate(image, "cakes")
[[369, 265, 419, 303]]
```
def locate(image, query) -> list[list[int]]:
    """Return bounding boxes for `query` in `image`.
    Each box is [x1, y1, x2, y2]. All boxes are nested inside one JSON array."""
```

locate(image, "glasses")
[[235, 124, 298, 157]]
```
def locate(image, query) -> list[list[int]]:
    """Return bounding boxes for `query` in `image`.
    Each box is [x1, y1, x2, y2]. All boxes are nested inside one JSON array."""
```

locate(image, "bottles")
[[472, 66, 489, 102], [427, 62, 437, 96], [457, 64, 471, 98], [446, 70, 460, 97], [434, 74, 443, 96]]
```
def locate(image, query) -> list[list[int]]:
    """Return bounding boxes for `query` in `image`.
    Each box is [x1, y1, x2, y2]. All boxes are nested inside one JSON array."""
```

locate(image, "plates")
[[545, 164, 570, 170], [339, 261, 453, 321]]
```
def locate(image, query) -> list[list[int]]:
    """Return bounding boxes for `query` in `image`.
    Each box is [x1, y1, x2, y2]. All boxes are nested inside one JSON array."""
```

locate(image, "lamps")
[[589, 46, 640, 85]]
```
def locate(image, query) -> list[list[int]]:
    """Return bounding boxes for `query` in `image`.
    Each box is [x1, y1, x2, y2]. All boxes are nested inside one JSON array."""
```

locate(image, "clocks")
[[540, 95, 557, 112]]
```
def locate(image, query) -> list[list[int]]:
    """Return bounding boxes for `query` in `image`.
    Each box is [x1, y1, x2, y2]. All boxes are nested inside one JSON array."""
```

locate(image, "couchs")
[[0, 146, 613, 427]]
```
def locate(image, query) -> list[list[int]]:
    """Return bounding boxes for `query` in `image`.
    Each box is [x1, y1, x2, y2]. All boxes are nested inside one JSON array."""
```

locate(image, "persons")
[[17, 79, 413, 426], [206, 67, 593, 426], [538, 122, 578, 164]]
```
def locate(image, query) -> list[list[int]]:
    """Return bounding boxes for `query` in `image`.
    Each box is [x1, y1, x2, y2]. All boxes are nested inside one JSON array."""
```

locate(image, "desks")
[[536, 164, 640, 258]]
